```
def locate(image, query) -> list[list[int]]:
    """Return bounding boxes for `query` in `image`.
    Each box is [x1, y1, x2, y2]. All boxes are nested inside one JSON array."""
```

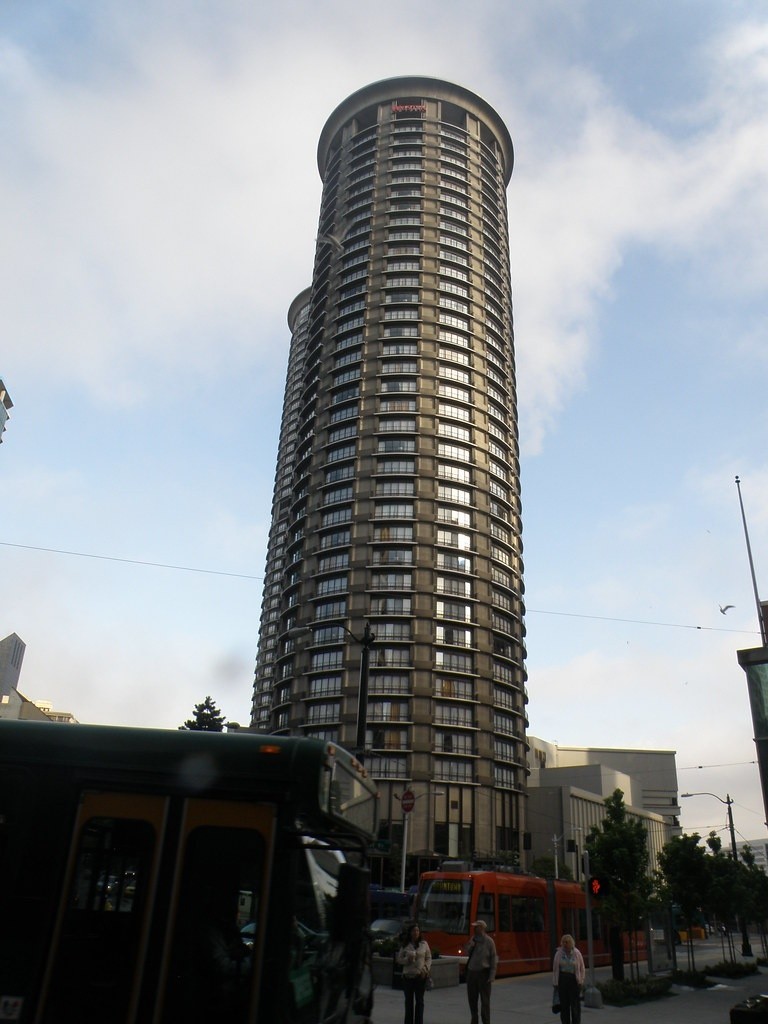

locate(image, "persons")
[[397, 922, 431, 1024], [553, 934, 586, 1024], [466, 920, 499, 1024]]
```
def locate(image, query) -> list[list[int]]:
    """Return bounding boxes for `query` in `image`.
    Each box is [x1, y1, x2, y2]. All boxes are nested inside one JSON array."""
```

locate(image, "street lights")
[[400, 790, 445, 892], [680, 791, 755, 957]]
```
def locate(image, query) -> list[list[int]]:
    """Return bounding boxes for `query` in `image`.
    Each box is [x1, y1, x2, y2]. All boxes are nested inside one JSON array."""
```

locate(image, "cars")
[[368, 916, 404, 946]]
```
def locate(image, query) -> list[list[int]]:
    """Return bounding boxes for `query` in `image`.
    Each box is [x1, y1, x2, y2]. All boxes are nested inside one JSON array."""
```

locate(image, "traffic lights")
[[588, 877, 605, 899]]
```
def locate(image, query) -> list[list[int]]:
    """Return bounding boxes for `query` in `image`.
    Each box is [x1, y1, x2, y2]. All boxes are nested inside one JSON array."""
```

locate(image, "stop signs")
[[401, 790, 416, 812]]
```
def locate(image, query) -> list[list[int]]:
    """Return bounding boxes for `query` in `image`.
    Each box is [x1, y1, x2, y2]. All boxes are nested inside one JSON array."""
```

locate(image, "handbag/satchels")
[[551, 989, 561, 1013], [426, 978, 434, 992], [464, 965, 468, 977]]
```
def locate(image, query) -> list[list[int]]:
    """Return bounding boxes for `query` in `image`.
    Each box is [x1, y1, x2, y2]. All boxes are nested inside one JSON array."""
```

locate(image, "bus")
[[411, 863, 648, 978], [0, 719, 392, 1023]]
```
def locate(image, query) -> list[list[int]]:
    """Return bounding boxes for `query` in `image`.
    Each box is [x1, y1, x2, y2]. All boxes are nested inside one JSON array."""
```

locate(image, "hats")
[[471, 920, 487, 928]]
